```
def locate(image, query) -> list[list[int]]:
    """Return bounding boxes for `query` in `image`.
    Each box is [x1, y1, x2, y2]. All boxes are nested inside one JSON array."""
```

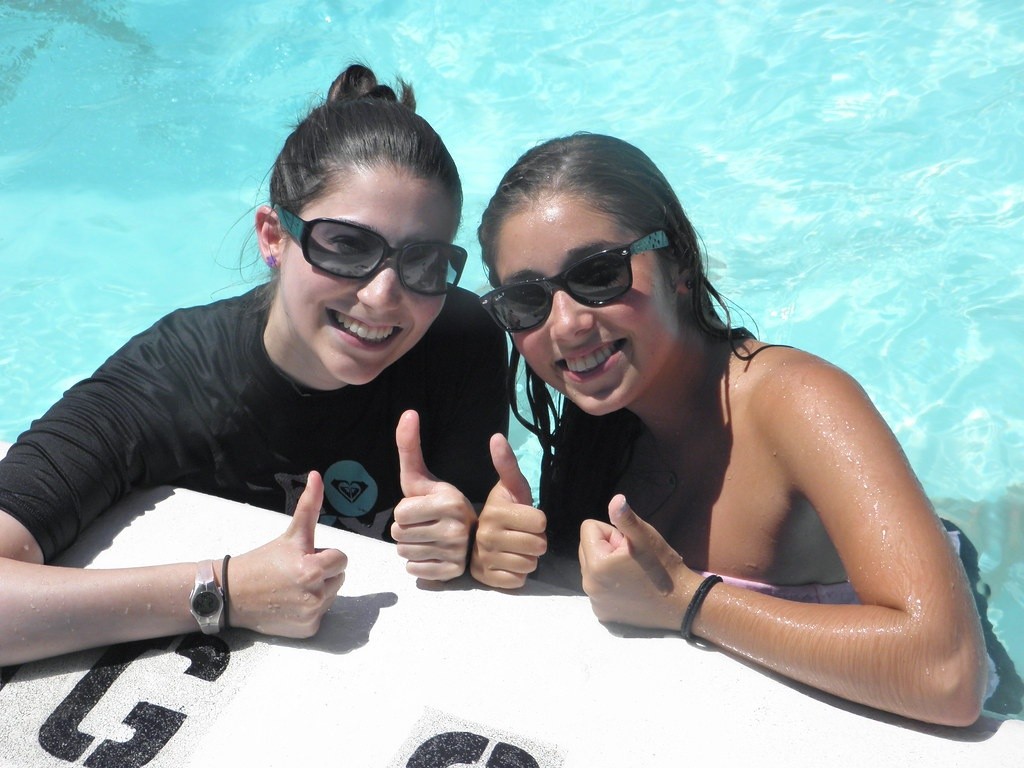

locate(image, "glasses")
[[478, 228, 670, 334], [272, 204, 468, 296]]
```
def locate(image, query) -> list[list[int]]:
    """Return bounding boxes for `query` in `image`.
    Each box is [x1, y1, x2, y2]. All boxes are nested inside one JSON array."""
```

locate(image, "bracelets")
[[679, 571, 725, 644], [221, 554, 231, 628]]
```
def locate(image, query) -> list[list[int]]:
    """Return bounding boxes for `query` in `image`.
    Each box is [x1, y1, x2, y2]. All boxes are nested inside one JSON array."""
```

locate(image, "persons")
[[0, 63, 510, 666], [470, 132, 989, 728]]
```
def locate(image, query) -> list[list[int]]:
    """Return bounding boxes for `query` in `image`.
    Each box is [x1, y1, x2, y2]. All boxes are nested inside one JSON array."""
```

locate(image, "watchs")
[[188, 558, 224, 636]]
